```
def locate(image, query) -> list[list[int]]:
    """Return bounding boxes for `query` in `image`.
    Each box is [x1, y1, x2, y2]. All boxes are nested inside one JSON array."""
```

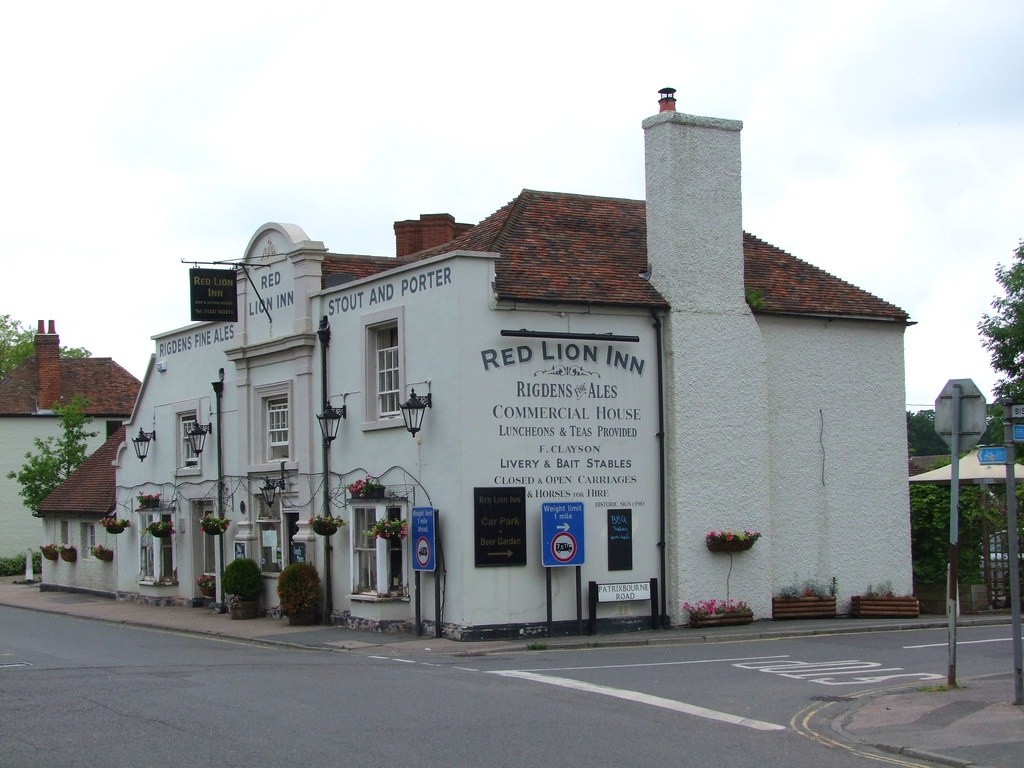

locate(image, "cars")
[[971, 529, 1024, 609]]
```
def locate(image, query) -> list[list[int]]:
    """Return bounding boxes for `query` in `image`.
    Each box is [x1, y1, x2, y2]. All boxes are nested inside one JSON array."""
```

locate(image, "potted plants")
[[772, 577, 838, 617]]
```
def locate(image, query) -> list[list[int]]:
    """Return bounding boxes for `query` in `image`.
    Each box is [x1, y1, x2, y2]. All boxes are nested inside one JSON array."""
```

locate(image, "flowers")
[[345, 472, 381, 498], [198, 575, 216, 588], [145, 521, 175, 535], [92, 545, 107, 556], [99, 517, 125, 527], [200, 513, 230, 534], [307, 515, 345, 528], [364, 518, 408, 542], [706, 528, 762, 544], [44, 544, 57, 553], [681, 596, 752, 617], [138, 492, 161, 509]]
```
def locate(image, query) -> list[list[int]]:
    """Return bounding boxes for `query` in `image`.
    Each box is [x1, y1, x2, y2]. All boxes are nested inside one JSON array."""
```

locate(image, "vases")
[[353, 485, 385, 498], [379, 530, 403, 541], [311, 520, 338, 536], [709, 540, 755, 553], [688, 613, 753, 626]]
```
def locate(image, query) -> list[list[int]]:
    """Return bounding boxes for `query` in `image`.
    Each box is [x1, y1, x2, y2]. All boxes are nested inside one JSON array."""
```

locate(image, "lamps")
[[398, 387, 432, 438], [186, 420, 213, 458], [131, 429, 155, 464], [259, 475, 284, 508], [315, 401, 346, 446]]
